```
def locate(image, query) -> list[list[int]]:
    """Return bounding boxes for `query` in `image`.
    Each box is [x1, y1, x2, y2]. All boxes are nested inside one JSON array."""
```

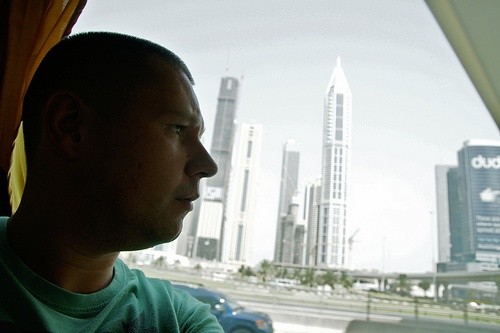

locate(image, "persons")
[[0, 31, 224, 333]]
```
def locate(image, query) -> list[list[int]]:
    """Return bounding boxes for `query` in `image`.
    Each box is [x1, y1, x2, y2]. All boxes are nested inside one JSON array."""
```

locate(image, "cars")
[[169, 279, 275, 333]]
